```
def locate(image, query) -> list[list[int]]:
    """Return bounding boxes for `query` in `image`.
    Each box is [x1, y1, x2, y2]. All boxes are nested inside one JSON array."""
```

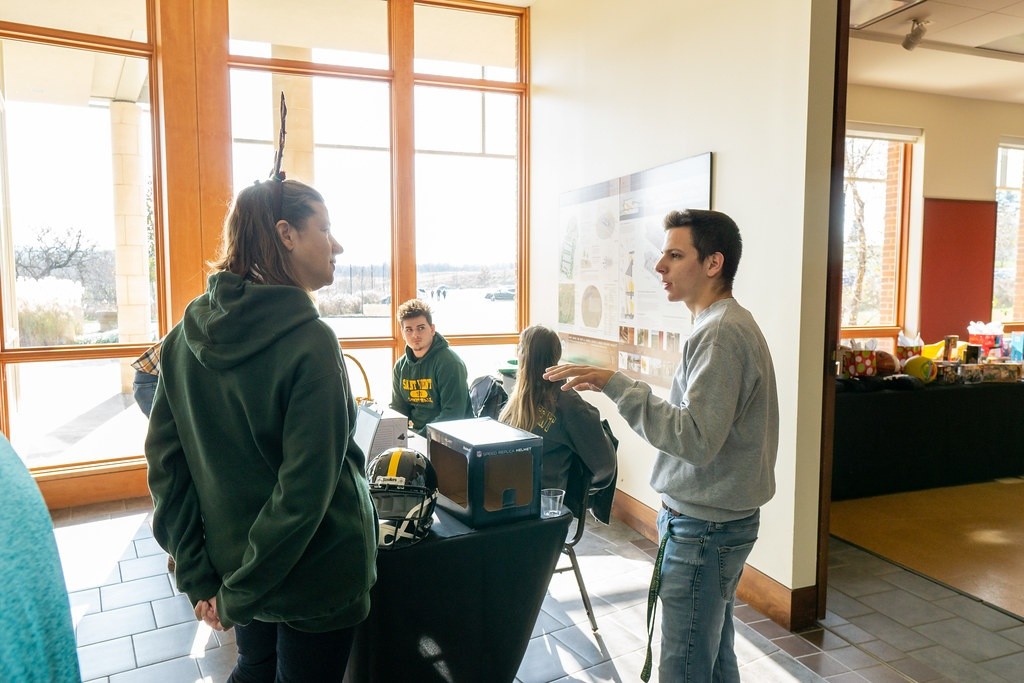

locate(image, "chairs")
[[553, 453, 598, 632], [469, 374, 509, 420]]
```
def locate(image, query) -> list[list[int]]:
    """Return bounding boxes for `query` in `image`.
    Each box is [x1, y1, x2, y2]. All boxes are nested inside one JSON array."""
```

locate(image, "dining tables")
[[832, 381, 1023, 501]]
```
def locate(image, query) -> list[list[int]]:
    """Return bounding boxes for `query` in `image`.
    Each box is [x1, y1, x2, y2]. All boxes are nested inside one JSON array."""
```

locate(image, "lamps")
[[901, 20, 926, 53]]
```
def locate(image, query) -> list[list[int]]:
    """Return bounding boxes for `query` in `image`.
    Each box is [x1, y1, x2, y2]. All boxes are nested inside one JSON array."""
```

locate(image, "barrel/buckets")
[[967, 333, 1005, 359]]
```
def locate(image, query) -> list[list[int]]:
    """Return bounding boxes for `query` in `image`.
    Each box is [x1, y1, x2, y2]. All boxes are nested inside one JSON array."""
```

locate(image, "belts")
[[660, 501, 680, 517]]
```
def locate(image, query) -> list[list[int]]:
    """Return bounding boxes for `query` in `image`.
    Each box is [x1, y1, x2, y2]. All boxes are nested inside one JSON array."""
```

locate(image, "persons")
[[542, 208, 781, 683], [430, 288, 447, 300], [496, 323, 618, 504], [387, 298, 475, 439], [144, 179, 381, 683], [0, 431, 83, 683], [130, 334, 168, 421]]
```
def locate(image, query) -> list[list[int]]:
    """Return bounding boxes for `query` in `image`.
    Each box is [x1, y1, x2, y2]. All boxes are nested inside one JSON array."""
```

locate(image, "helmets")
[[366, 447, 439, 550]]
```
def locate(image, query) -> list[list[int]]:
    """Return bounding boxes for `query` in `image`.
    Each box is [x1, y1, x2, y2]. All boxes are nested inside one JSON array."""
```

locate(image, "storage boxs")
[[426, 415, 544, 528]]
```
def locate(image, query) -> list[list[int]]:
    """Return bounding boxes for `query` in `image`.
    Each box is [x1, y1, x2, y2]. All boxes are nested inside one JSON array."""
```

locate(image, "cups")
[[943, 335, 958, 361], [843, 349, 876, 377], [541, 488, 565, 518], [966, 345, 981, 362], [896, 346, 921, 373], [1010, 330, 1023, 361]]
